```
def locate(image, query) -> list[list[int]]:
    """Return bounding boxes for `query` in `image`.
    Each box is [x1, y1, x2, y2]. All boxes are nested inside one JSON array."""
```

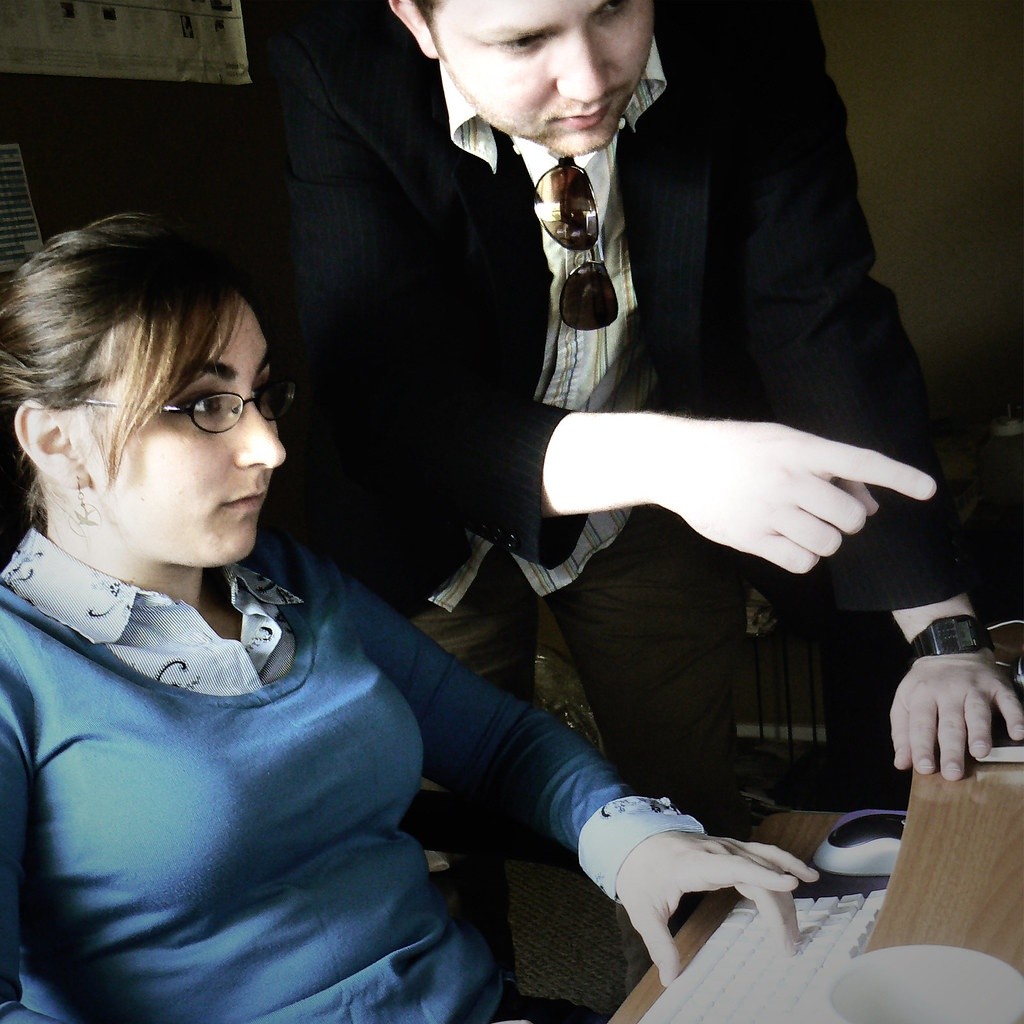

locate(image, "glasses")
[[75, 378, 296, 435], [532, 157, 620, 332]]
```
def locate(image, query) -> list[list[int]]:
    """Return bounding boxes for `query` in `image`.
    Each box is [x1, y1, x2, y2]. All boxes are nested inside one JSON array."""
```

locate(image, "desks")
[[604, 762, 1024, 1024]]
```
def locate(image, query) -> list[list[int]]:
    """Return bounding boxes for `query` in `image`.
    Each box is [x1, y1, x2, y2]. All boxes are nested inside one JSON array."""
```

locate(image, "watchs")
[[905, 614, 996, 661]]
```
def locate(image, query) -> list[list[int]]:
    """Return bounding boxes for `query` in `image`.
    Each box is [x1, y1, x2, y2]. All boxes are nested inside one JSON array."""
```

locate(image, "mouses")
[[813, 814, 910, 875]]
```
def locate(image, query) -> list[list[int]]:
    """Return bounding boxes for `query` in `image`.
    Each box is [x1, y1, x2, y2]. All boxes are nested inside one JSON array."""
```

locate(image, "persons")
[[276, 1, 1024, 999], [0, 213, 822, 1024]]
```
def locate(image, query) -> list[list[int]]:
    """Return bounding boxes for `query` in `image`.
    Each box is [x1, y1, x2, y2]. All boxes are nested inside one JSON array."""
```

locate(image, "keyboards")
[[636, 888, 890, 1024]]
